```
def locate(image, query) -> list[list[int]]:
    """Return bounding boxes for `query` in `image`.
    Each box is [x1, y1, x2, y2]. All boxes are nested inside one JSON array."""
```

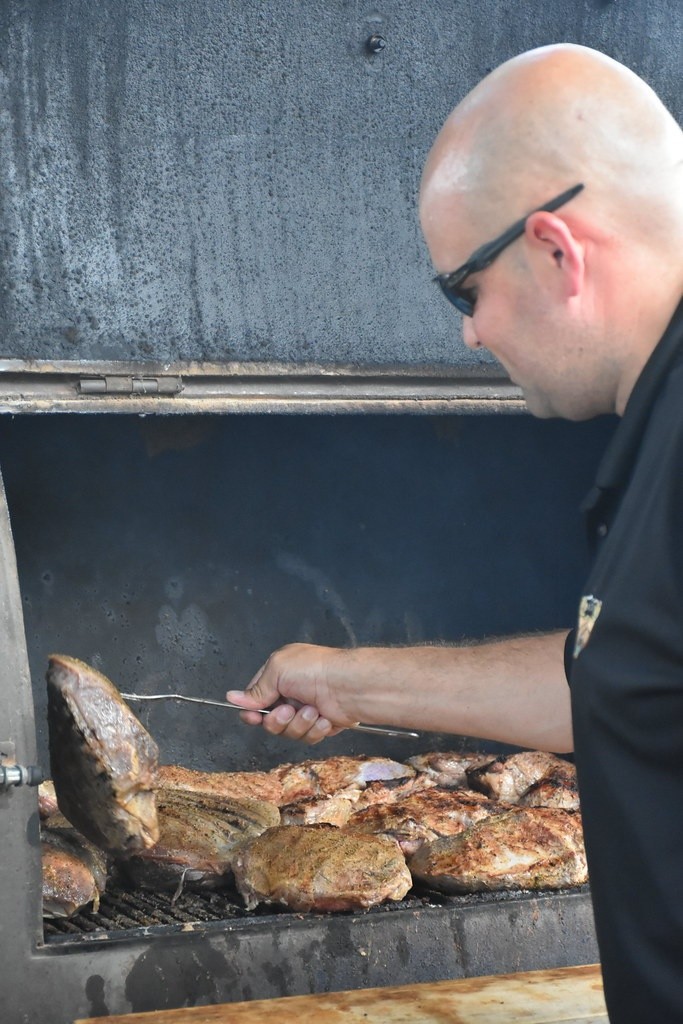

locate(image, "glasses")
[[428, 184, 582, 320]]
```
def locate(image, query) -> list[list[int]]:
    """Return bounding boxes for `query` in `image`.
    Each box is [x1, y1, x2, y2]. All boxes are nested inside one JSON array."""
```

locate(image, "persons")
[[228, 43, 683, 1024]]
